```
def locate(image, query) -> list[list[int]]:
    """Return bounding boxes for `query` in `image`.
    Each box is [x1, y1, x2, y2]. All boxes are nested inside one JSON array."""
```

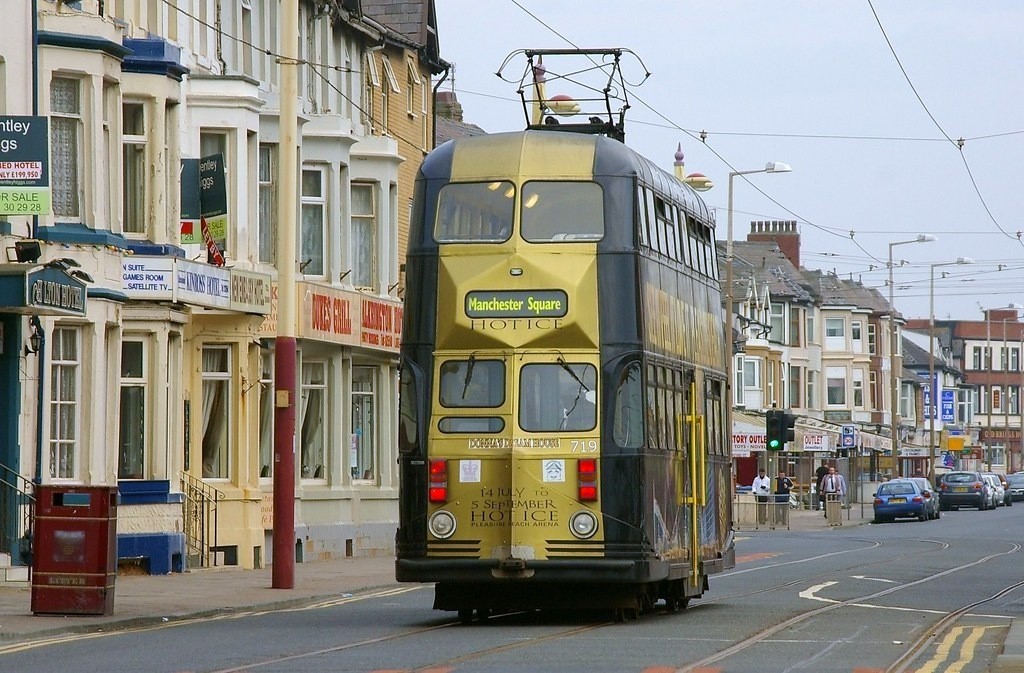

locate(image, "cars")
[[980, 473, 1012, 509], [872, 482, 928, 522], [889, 478, 941, 519], [1006, 474, 1024, 501]]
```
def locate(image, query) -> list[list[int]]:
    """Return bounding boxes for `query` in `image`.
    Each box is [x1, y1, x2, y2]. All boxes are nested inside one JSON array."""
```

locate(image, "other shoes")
[[782, 523, 786, 525], [775, 519, 780, 524], [759, 518, 767, 524], [816, 507, 819, 510]]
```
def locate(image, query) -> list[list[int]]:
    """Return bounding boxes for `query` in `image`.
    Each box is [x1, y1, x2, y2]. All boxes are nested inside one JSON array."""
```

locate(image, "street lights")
[[889, 234, 938, 478], [724, 161, 793, 522], [529, 54, 581, 131], [929, 257, 976, 489], [674, 142, 716, 193], [986, 303, 1024, 472]]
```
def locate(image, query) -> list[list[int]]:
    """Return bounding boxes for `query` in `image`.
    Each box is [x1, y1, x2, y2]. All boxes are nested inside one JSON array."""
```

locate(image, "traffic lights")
[[782, 410, 798, 451], [767, 411, 782, 451]]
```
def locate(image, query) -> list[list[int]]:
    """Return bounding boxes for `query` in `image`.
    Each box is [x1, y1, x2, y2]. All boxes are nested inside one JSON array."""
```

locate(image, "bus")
[[396, 126, 739, 622], [940, 471, 989, 511]]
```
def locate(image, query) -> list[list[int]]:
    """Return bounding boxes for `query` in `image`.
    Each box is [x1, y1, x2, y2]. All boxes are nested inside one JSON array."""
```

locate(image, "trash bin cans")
[[31, 484, 118, 617], [826, 493, 842, 527]]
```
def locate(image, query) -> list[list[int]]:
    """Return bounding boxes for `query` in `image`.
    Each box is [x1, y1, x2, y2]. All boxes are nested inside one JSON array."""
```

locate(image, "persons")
[[814, 463, 848, 517], [752, 468, 770, 523], [914, 469, 924, 478], [774, 472, 794, 524]]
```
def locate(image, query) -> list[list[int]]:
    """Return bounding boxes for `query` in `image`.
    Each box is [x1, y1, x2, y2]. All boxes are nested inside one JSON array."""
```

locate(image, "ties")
[[831, 475, 834, 489]]
